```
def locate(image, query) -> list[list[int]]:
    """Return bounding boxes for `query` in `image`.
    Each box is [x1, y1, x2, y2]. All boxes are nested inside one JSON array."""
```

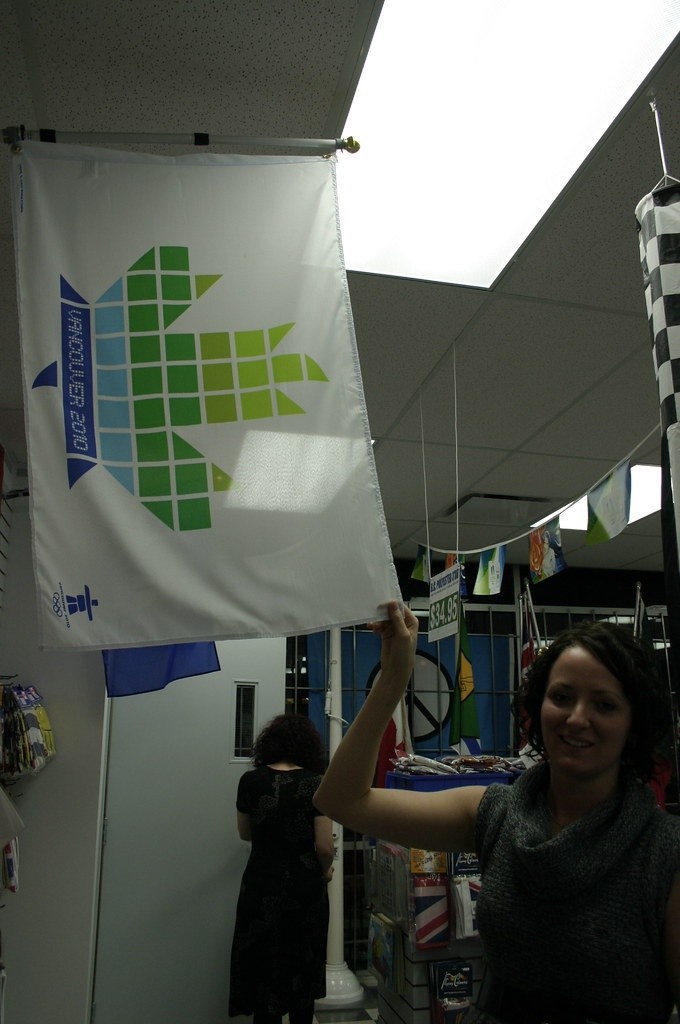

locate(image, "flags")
[[378, 690, 414, 789], [632, 589, 655, 650], [517, 593, 541, 753], [449, 597, 481, 745], [6, 144, 403, 652]]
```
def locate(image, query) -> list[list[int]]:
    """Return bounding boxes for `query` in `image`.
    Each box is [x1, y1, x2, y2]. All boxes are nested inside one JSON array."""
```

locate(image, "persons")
[[227, 714, 334, 1024], [315, 604, 680, 1024]]
